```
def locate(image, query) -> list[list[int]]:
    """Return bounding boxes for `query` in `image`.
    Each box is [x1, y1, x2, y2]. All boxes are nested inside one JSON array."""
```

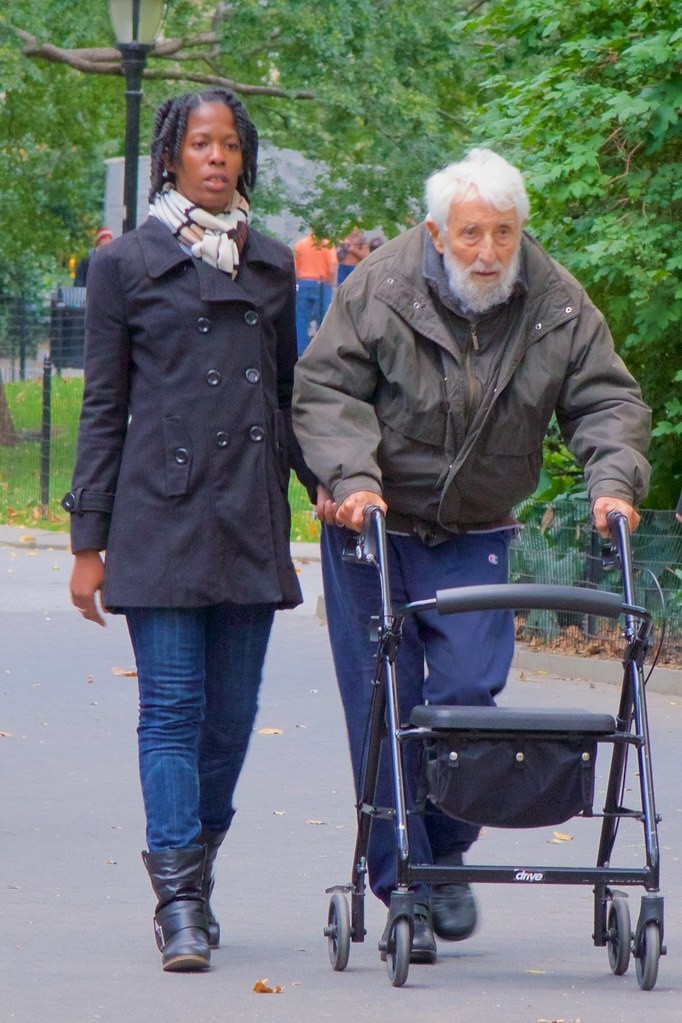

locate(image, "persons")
[[73, 227, 112, 288], [294, 226, 384, 360], [61, 90, 344, 973], [290, 148, 656, 964]]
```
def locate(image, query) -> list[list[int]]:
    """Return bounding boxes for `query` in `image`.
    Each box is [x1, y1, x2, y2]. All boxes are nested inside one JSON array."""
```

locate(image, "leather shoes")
[[430, 853, 477, 941], [412, 897, 438, 962]]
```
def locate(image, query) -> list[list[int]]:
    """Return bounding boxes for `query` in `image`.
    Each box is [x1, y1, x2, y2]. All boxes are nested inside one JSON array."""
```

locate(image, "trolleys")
[[321, 505, 668, 992]]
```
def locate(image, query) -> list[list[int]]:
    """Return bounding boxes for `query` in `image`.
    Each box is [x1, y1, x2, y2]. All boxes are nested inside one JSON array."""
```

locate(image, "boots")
[[201, 830, 227, 948], [141, 844, 211, 971]]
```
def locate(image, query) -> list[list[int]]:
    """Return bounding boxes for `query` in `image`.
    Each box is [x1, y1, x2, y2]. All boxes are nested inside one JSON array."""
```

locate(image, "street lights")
[[106, 0, 165, 237]]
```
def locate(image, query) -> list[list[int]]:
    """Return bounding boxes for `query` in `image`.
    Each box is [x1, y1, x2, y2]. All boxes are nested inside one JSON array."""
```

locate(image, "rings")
[[79, 608, 87, 613]]
